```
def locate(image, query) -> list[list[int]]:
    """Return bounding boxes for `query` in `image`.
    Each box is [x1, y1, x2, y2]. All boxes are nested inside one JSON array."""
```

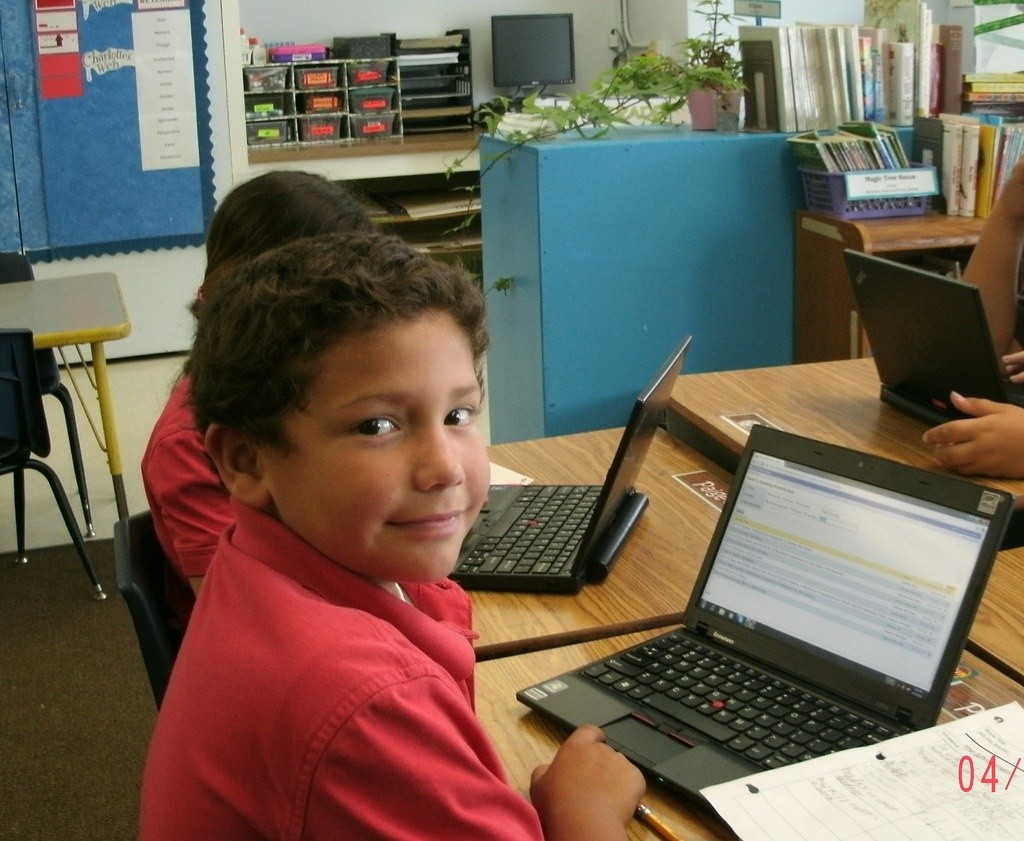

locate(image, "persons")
[[141, 229, 648, 841], [923, 148, 1024, 476], [138, 170, 380, 653]]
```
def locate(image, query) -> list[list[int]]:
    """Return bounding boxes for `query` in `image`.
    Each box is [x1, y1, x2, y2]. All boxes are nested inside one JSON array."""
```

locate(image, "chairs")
[[0, 253, 96, 538], [114, 509, 197, 712], [0, 328, 108, 603]]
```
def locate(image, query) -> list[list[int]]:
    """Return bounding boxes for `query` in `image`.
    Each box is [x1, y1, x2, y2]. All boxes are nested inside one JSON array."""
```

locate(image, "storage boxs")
[[350, 114, 396, 139], [295, 67, 339, 89], [349, 87, 395, 114], [244, 95, 283, 120], [246, 121, 287, 145], [269, 45, 325, 62], [347, 62, 389, 86], [334, 36, 390, 59], [298, 116, 343, 142], [302, 91, 343, 114], [244, 67, 288, 91]]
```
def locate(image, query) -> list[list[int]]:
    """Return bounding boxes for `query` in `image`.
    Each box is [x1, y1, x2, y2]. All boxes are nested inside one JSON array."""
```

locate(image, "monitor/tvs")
[[492, 13, 575, 102]]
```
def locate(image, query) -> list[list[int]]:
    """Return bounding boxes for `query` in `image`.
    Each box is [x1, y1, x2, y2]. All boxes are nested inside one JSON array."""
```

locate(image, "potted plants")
[[444, 39, 750, 236]]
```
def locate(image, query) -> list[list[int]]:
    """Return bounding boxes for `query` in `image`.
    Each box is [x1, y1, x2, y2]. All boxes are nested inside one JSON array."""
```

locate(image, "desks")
[[468, 425, 735, 651], [792, 210, 988, 362], [968, 547, 1024, 676], [665, 356, 1024, 550], [475, 625, 1024, 841], [0, 272, 130, 520]]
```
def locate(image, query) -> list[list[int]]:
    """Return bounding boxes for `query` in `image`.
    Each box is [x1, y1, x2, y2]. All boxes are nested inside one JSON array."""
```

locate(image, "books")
[[739, 1, 1024, 216]]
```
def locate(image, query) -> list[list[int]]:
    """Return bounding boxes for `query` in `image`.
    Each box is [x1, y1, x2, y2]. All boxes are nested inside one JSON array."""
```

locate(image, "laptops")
[[514, 423, 1017, 816], [447, 334, 693, 595], [841, 249, 1024, 427]]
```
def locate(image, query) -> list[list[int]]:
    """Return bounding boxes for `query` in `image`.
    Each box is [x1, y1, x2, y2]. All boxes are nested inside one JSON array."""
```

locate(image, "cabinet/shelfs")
[[242, 56, 403, 151], [380, 29, 475, 135]]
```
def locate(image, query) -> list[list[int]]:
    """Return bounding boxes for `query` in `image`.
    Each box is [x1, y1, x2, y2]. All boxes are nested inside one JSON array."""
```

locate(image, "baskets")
[[796, 163, 932, 220]]
